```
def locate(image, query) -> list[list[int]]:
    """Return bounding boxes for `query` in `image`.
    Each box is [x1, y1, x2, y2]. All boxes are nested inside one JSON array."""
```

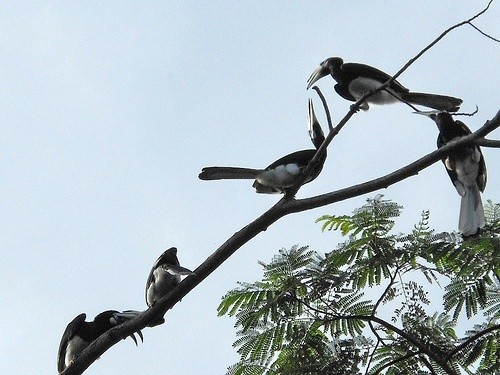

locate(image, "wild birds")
[[197, 98, 327, 197], [111, 246, 197, 328], [306, 57, 463, 113], [56, 310, 144, 374], [411, 108, 488, 237]]
